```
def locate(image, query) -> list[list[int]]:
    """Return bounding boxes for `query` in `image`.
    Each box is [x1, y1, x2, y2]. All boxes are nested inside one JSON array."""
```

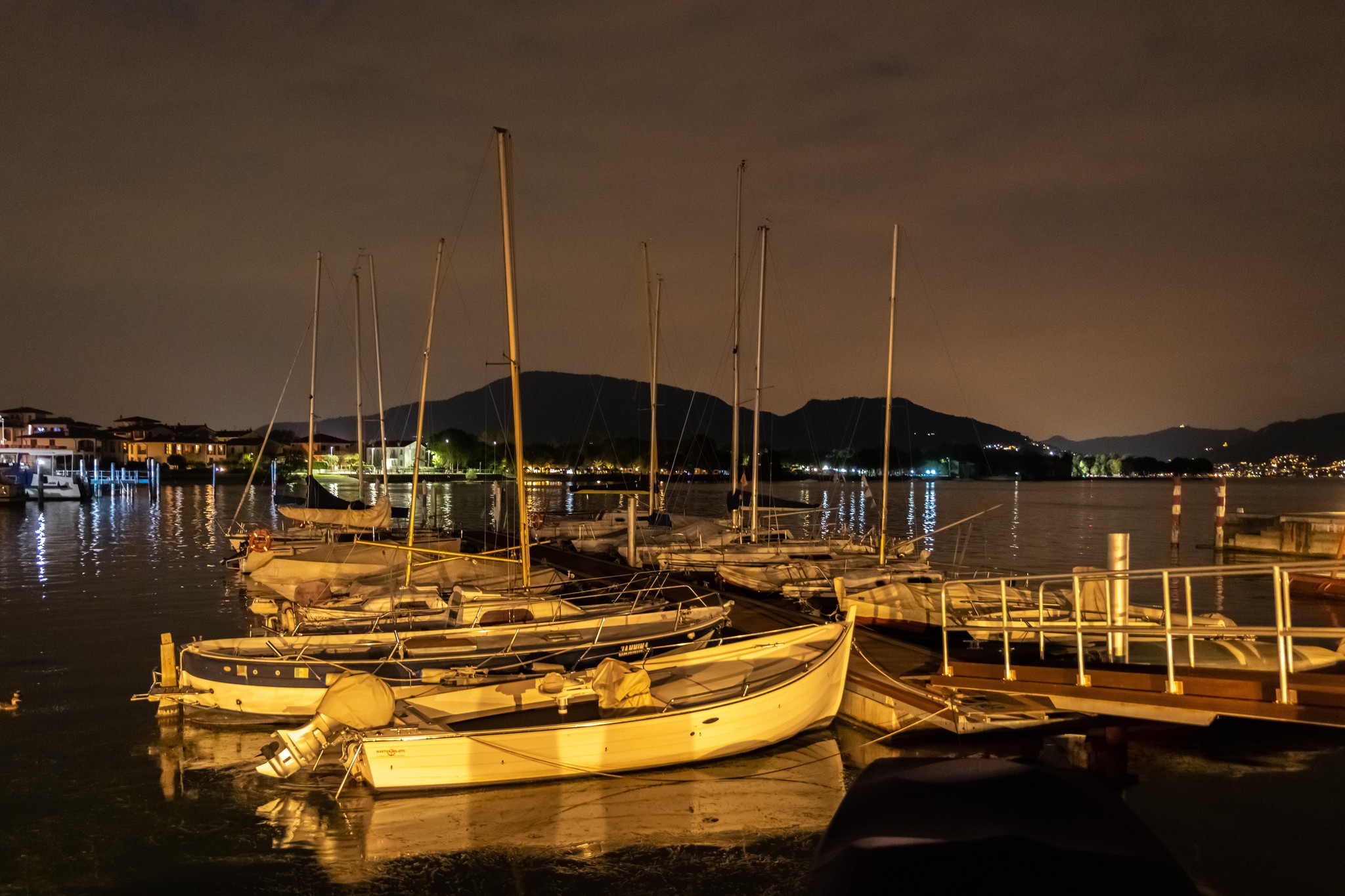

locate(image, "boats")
[[0, 447, 95, 507], [248, 729, 849, 887]]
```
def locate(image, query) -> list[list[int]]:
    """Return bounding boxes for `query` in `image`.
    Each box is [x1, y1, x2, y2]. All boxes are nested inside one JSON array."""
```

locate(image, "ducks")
[[0, 693, 22, 711]]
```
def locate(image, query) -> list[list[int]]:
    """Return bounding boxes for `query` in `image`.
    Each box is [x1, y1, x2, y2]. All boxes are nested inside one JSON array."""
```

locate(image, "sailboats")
[[130, 122, 1344, 793]]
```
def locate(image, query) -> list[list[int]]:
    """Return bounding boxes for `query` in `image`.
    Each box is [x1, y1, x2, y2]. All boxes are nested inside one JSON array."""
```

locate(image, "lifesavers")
[[249, 530, 271, 553], [532, 513, 543, 530]]
